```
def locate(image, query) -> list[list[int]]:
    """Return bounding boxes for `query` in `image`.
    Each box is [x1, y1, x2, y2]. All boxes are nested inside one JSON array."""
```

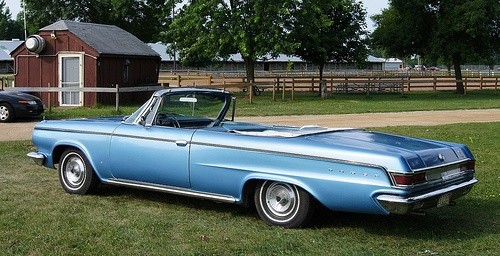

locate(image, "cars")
[[404, 64, 439, 71], [23, 87, 476, 228], [0, 89, 43, 121]]
